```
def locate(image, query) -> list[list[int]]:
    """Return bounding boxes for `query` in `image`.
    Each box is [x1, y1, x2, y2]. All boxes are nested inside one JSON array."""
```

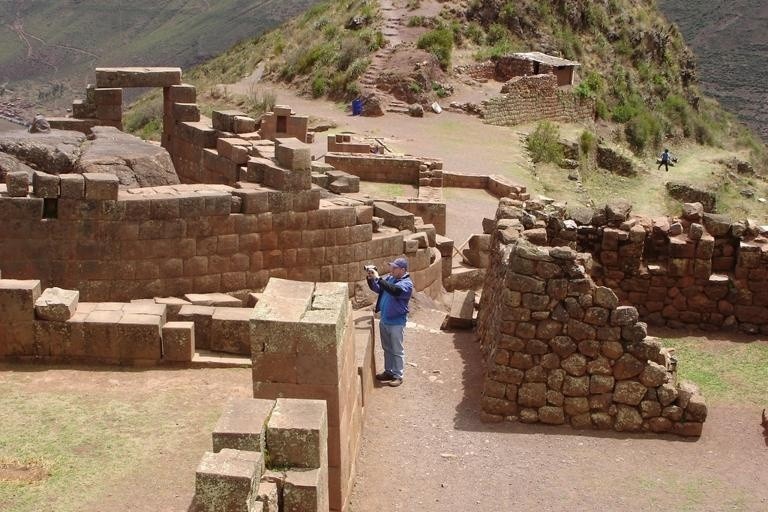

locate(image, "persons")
[[364, 258, 414, 387], [658, 149, 670, 172]]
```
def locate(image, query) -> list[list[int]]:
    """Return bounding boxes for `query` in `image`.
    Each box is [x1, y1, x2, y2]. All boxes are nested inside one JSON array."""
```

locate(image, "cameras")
[[364, 265, 378, 275]]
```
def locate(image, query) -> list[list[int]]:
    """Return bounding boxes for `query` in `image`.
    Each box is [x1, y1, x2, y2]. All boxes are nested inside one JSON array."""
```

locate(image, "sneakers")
[[376, 371, 403, 386]]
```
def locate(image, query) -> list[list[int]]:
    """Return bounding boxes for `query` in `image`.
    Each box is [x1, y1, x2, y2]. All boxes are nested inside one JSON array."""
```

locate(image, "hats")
[[388, 258, 408, 268]]
